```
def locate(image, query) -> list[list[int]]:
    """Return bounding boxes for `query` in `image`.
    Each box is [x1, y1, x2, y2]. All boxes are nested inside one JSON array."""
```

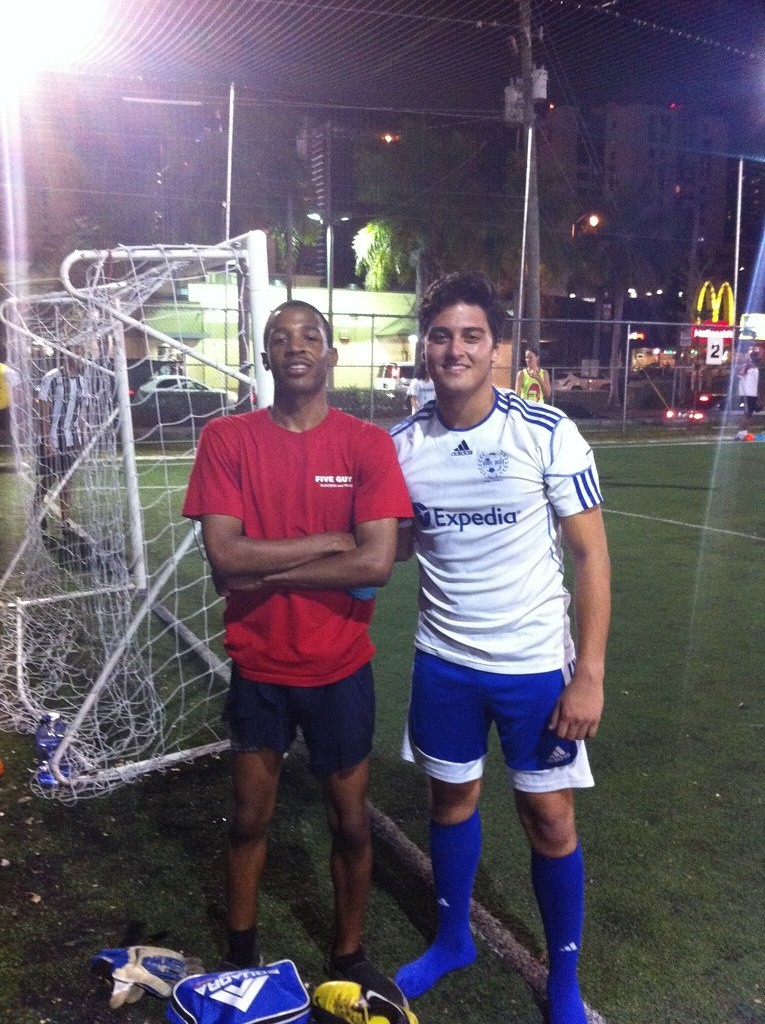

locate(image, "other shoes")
[[42, 533, 55, 543], [62, 522, 71, 533]]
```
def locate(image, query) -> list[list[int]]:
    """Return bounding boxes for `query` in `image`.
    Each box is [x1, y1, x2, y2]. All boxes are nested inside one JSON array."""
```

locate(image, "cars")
[[112, 374, 238, 427], [548, 371, 612, 392], [664, 394, 765, 423]]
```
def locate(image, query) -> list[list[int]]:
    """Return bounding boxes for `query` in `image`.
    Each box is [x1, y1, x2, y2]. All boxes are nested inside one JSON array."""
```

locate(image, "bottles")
[[38, 712, 73, 784]]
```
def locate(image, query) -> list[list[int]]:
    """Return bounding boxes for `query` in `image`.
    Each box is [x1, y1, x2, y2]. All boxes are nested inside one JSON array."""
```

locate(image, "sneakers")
[[323, 950, 409, 1010], [212, 953, 265, 973]]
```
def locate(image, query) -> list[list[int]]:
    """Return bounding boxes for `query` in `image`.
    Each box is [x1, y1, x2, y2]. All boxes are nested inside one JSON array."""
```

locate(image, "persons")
[[32, 345, 90, 532], [181, 300, 415, 1009], [388, 268, 611, 1024], [513, 348, 553, 405], [406, 360, 437, 416], [738, 352, 761, 431]]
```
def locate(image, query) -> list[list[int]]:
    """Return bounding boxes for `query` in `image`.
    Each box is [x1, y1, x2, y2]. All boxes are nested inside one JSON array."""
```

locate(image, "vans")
[[373, 361, 416, 399]]
